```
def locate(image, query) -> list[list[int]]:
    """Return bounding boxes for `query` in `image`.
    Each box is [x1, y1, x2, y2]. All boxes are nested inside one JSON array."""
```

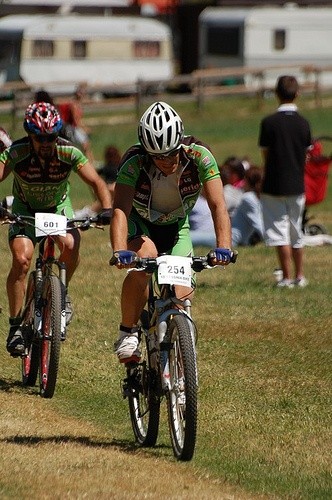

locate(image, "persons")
[[258, 76, 313, 289], [110, 102, 231, 405], [94, 139, 332, 248], [0, 102, 111, 356], [32, 91, 89, 154]]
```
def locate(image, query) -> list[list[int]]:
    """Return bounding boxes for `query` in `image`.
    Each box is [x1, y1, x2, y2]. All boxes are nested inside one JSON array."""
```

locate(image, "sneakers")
[[147, 149, 181, 161], [5, 317, 26, 356], [63, 291, 73, 325], [175, 388, 188, 414], [114, 327, 143, 364]]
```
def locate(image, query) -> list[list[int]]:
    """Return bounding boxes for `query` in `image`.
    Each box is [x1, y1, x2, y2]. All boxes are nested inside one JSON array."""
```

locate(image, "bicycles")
[[109, 248, 240, 462], [1, 206, 114, 399]]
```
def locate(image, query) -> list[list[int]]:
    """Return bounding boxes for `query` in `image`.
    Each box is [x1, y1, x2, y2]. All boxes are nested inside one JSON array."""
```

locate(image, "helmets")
[[23, 103, 62, 134], [137, 101, 185, 153]]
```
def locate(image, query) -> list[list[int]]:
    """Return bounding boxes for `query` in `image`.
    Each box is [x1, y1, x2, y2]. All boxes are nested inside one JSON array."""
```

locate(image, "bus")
[[197, 7, 332, 87], [1, 14, 174, 98]]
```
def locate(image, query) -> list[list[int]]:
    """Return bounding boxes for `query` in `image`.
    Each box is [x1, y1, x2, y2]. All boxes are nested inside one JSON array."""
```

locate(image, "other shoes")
[[295, 278, 308, 288], [277, 279, 293, 288]]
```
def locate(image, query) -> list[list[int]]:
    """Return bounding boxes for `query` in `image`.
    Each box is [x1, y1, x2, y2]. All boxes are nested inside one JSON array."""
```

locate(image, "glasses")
[[33, 132, 58, 142]]
